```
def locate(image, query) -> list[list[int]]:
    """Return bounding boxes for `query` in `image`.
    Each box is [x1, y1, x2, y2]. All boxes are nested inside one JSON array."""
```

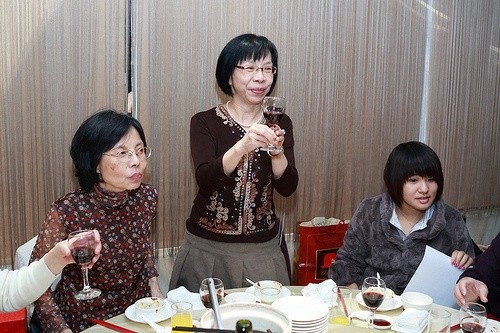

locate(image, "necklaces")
[[226, 101, 264, 128]]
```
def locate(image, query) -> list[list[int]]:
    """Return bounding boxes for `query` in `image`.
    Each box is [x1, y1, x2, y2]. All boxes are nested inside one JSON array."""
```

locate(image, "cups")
[[199, 279, 224, 310], [260, 288, 280, 304], [332, 287, 351, 326], [255, 280, 282, 301], [427, 308, 451, 333], [460, 302, 487, 333], [135, 297, 163, 318], [171, 302, 193, 333]]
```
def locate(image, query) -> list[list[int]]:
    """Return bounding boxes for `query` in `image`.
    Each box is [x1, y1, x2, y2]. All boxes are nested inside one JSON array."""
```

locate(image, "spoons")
[[139, 315, 172, 333]]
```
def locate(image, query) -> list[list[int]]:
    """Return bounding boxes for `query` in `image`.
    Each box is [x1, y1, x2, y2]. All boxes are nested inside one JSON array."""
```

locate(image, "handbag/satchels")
[[293, 217, 350, 286]]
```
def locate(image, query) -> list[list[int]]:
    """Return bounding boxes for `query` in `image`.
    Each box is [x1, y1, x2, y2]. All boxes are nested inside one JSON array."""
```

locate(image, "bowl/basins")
[[201, 304, 289, 333], [400, 292, 433, 311]]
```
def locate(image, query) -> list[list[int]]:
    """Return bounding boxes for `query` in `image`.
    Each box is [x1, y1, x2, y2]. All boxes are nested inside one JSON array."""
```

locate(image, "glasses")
[[100, 144, 153, 160], [235, 65, 277, 75]]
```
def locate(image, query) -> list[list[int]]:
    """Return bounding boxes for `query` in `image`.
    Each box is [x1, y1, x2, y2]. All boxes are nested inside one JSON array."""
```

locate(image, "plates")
[[356, 292, 402, 311], [366, 314, 393, 329], [246, 286, 291, 303], [225, 292, 256, 303], [272, 297, 329, 333], [125, 301, 176, 324]]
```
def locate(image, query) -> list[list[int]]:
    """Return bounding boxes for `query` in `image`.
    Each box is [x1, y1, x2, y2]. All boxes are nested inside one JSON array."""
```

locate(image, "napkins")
[[301, 279, 338, 308], [166, 286, 200, 310], [397, 307, 430, 332]]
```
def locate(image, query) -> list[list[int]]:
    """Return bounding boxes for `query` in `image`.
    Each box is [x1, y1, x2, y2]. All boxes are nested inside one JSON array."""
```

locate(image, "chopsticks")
[[91, 319, 137, 333], [173, 327, 265, 333], [449, 324, 460, 332]]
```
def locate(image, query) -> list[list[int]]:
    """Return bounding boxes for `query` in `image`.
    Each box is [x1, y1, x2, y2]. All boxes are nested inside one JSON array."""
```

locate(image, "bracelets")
[[267, 149, 284, 158]]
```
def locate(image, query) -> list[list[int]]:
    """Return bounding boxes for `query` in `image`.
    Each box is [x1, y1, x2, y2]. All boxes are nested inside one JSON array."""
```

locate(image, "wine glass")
[[260, 97, 287, 151], [69, 231, 101, 300], [362, 277, 386, 329]]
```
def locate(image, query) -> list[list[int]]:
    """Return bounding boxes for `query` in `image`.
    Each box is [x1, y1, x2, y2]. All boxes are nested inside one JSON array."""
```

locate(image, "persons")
[[328, 141, 482, 298], [454, 232, 500, 319], [0, 230, 101, 313], [168, 34, 299, 292], [28, 107, 165, 333]]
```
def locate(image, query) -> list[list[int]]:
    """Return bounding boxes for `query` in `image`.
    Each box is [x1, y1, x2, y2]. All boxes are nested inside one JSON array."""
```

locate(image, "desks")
[[80, 286, 500, 333]]
